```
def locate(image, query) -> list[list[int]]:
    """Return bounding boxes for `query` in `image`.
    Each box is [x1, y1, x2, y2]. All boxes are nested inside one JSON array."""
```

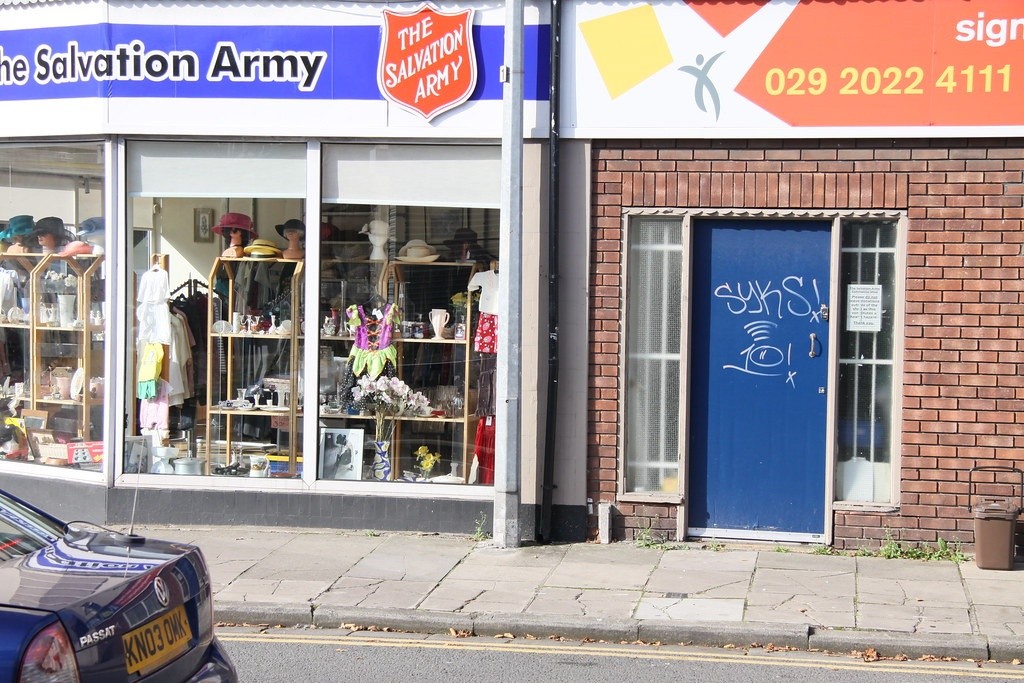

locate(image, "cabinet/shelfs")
[[205, 199, 481, 485], [0, 253, 137, 443]]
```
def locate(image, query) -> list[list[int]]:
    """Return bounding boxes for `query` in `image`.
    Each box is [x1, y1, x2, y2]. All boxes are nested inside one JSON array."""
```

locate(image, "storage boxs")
[[266, 455, 303, 477]]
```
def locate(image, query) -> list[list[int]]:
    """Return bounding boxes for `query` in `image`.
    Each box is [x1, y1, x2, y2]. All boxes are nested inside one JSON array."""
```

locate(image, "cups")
[[238, 389, 247, 400], [250, 455, 270, 478]]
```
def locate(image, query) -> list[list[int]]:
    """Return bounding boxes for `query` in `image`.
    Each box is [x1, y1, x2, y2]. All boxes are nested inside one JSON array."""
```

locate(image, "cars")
[[0, 493, 237, 682]]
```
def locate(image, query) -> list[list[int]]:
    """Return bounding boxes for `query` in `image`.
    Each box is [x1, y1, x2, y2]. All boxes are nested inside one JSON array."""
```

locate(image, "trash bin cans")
[[973, 501, 1018, 571]]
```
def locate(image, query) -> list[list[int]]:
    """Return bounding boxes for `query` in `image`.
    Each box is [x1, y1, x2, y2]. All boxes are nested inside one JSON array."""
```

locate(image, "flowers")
[[352, 375, 431, 440], [45, 268, 77, 294], [414, 445, 442, 480]]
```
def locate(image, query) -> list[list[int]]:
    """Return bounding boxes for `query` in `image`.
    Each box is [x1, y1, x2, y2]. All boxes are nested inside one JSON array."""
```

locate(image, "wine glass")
[[436, 385, 462, 418], [430, 308, 450, 340]]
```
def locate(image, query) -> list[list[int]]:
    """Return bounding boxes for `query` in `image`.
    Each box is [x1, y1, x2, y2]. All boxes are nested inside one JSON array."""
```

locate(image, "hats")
[[274, 219, 305, 242], [243, 239, 282, 255], [0, 214, 75, 248], [336, 434, 347, 444], [393, 228, 482, 262], [211, 213, 257, 240], [358, 219, 397, 241]]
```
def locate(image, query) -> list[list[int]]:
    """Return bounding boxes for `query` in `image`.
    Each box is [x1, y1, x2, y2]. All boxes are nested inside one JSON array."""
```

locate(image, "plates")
[[417, 414, 436, 417]]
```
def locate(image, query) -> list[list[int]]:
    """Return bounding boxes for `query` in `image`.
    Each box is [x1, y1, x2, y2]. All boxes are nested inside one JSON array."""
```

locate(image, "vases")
[[58, 293, 76, 329], [373, 441, 393, 482]]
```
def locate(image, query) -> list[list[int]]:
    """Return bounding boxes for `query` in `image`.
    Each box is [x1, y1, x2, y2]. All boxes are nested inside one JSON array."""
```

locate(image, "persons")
[[0, 215, 66, 255], [368, 219, 389, 260], [212, 212, 258, 249], [275, 219, 305, 259]]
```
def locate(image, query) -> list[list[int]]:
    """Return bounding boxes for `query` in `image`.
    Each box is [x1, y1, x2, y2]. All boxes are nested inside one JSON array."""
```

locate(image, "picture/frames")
[[194, 207, 213, 242], [316, 426, 366, 480], [423, 206, 468, 245]]
